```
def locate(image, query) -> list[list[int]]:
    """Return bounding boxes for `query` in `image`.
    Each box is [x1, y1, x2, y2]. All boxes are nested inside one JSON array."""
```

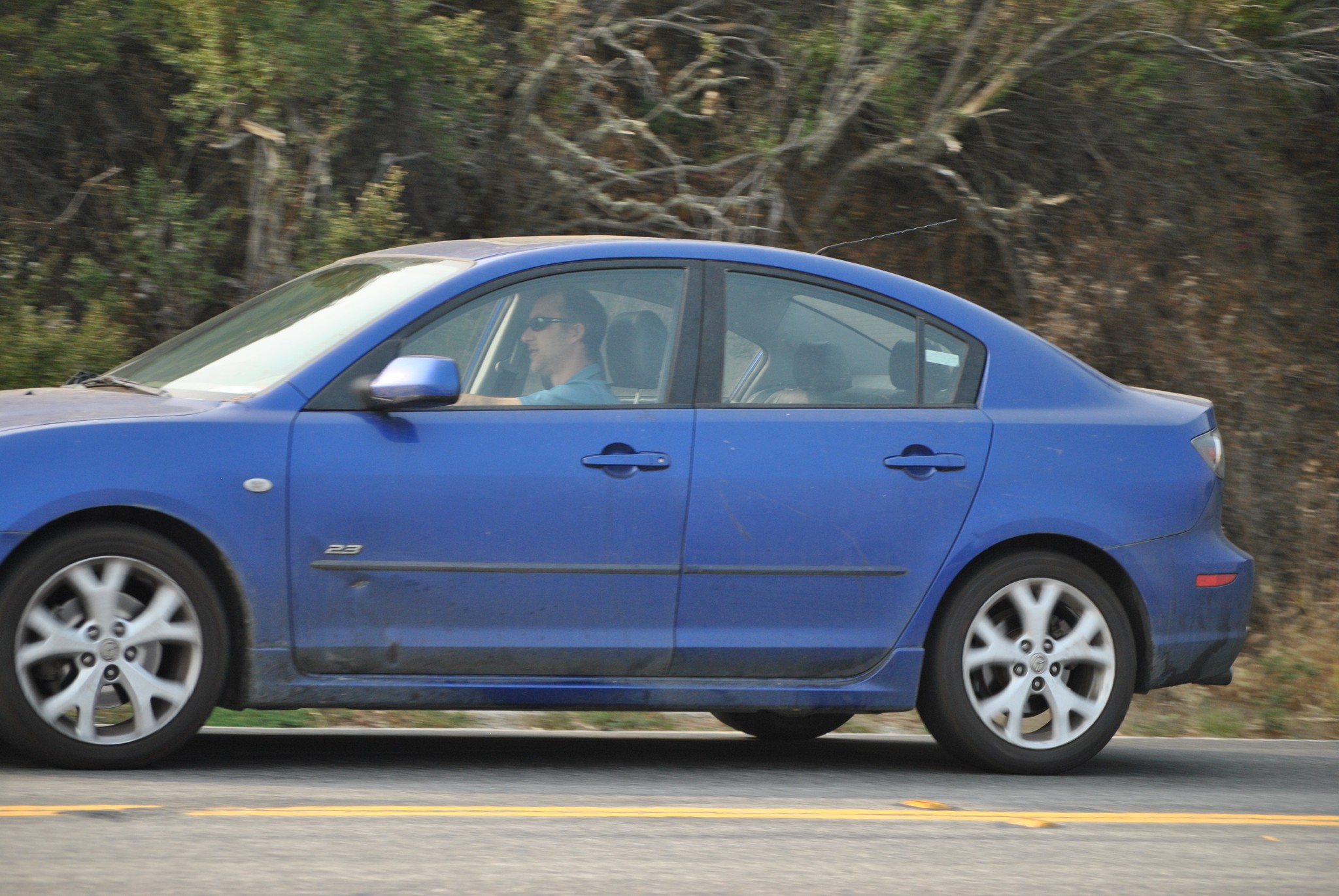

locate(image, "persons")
[[452, 285, 619, 406]]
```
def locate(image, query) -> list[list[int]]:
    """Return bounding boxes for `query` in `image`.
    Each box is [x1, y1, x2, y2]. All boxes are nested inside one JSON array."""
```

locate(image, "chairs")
[[605, 310, 667, 404], [889, 339, 952, 403], [764, 342, 852, 404]]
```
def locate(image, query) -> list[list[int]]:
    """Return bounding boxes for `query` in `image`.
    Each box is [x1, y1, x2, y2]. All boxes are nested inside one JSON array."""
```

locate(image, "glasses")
[[520, 315, 584, 332]]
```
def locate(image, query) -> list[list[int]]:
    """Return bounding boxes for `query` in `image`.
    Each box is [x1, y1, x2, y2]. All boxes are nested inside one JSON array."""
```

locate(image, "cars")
[[1, 231, 1257, 775]]
